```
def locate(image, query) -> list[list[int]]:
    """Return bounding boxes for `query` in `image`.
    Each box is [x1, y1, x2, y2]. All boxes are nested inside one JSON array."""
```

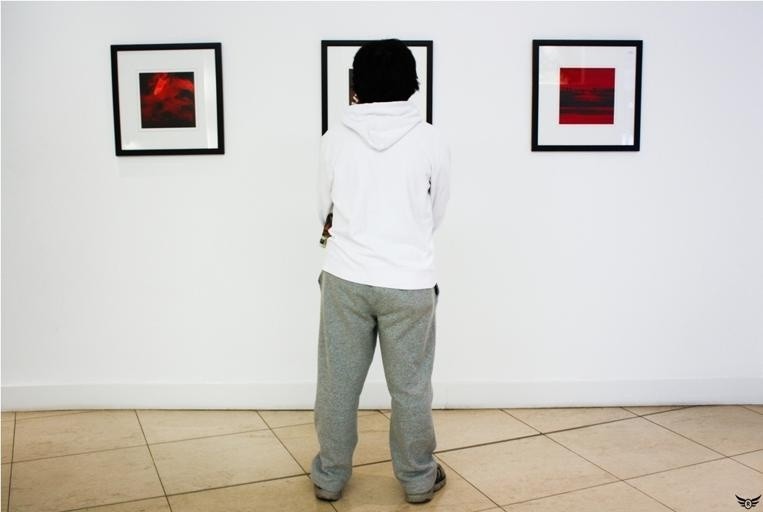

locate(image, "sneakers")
[[399, 459, 450, 505], [309, 482, 344, 504]]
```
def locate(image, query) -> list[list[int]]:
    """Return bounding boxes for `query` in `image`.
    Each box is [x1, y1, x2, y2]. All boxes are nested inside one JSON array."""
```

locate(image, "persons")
[[296, 39, 451, 506]]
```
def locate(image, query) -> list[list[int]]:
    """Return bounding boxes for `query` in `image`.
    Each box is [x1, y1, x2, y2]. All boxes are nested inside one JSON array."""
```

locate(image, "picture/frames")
[[318, 37, 434, 139], [529, 36, 643, 154], [109, 40, 228, 159]]
